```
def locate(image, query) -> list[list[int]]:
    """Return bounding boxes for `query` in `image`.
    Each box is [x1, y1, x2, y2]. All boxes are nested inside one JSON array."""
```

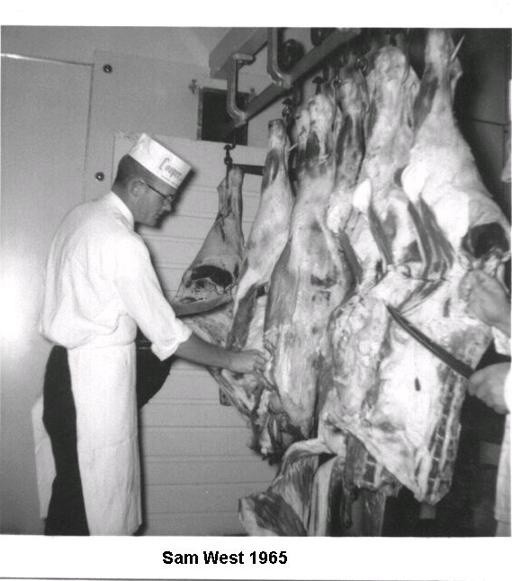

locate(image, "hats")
[[127, 133, 191, 189]]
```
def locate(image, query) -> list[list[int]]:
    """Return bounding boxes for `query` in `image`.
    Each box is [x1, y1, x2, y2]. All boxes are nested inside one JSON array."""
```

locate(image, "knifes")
[[384, 302, 473, 378], [171, 297, 225, 316]]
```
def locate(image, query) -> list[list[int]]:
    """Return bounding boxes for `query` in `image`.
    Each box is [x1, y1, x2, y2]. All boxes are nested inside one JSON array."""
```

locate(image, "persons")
[[459, 79, 511, 537], [38, 133, 266, 536]]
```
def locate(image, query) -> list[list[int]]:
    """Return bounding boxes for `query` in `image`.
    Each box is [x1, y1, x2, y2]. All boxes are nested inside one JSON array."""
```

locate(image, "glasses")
[[148, 183, 175, 206]]
